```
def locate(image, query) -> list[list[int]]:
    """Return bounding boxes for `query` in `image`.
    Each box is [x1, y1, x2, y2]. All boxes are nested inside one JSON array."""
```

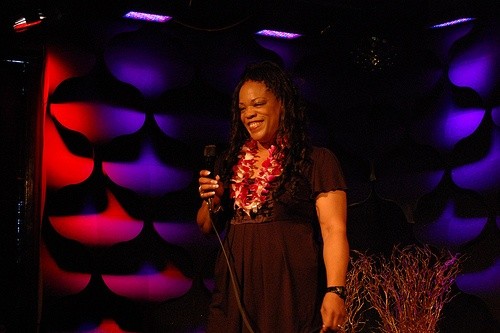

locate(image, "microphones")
[[203, 145, 216, 211]]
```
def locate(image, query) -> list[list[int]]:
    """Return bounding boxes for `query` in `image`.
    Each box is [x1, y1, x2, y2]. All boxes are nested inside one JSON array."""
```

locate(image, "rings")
[[337, 324, 341, 327], [201, 193, 203, 199], [199, 185, 202, 192]]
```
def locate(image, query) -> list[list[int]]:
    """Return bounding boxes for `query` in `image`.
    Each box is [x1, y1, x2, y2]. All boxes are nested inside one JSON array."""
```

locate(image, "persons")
[[197, 60, 350, 333]]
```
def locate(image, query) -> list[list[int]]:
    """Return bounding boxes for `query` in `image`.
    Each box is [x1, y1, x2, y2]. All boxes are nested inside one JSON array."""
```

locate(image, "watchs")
[[326, 286, 348, 301]]
